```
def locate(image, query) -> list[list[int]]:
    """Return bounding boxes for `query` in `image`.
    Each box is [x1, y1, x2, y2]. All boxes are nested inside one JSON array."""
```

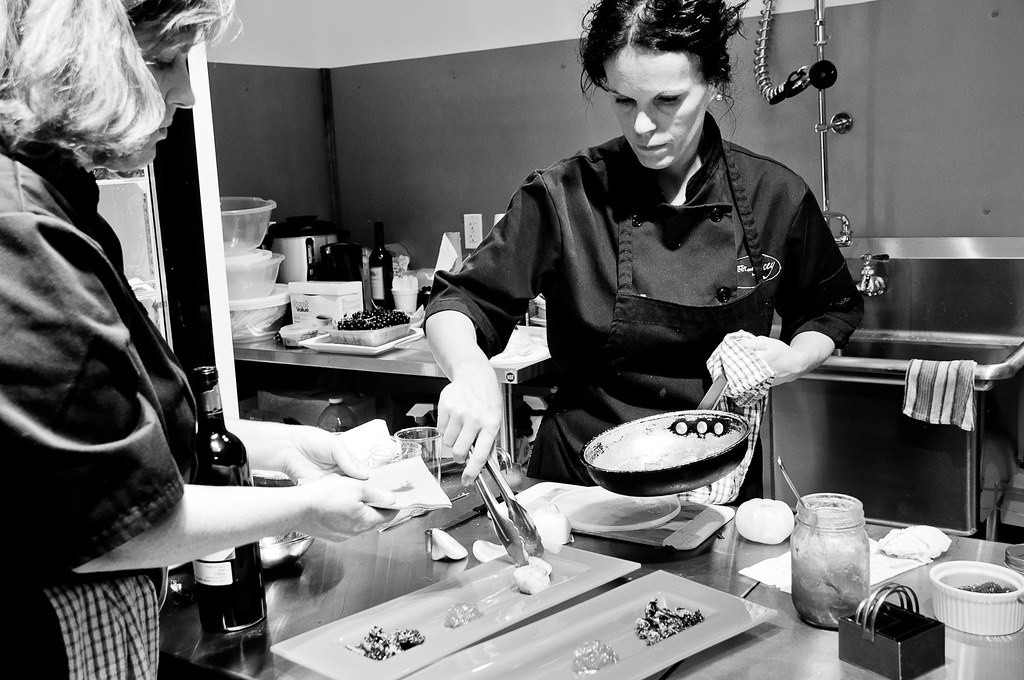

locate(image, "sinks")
[[829, 335, 1021, 367]]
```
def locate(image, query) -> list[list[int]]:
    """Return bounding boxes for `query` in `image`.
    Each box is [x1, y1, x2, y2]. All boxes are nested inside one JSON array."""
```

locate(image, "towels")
[[666, 328, 779, 506], [899, 357, 979, 433]]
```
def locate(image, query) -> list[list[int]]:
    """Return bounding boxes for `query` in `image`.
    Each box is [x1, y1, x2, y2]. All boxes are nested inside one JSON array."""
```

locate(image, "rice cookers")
[[261, 214, 339, 282]]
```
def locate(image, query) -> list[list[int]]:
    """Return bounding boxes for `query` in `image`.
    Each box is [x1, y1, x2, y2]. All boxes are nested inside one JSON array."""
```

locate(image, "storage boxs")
[[226, 252, 286, 303], [221, 196, 277, 253], [234, 281, 292, 343], [288, 276, 363, 329]]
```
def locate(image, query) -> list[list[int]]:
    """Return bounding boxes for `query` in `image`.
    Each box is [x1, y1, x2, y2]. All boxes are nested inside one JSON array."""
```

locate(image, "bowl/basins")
[[323, 322, 411, 347], [260, 531, 316, 573], [228, 283, 290, 344], [279, 323, 319, 349], [220, 197, 276, 255], [225, 251, 286, 299], [929, 561, 1024, 635]]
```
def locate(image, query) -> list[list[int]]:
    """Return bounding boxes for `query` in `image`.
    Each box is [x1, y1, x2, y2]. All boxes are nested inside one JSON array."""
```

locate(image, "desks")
[[239, 323, 552, 465]]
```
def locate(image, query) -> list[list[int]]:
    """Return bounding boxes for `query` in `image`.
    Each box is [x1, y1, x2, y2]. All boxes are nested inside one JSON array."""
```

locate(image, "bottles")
[[316, 397, 358, 434], [790, 493, 871, 631], [368, 220, 395, 310], [187, 367, 267, 632]]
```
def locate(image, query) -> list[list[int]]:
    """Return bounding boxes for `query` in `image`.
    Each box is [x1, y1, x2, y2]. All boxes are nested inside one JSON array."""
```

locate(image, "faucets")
[[854, 253, 890, 297]]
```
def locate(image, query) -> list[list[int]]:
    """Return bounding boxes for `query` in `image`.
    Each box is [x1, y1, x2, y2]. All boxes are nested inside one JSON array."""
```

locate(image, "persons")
[[0, 0, 397, 680], [422, 0, 865, 507]]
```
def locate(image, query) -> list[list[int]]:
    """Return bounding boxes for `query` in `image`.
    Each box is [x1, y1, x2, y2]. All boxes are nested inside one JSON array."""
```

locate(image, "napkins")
[[738, 538, 925, 592]]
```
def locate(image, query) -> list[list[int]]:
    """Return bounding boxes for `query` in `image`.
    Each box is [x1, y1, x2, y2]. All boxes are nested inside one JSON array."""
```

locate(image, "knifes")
[[439, 491, 518, 529]]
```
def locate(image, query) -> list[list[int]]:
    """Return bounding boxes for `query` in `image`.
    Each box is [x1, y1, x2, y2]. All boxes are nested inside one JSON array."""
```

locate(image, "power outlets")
[[463, 214, 484, 250]]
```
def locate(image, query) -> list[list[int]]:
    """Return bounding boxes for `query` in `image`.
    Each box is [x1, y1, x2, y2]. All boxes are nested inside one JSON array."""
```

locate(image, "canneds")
[[791, 492, 871, 630]]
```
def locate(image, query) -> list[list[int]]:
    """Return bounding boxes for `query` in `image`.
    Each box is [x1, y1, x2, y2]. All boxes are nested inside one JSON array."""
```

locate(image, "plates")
[[268, 541, 642, 680], [398, 568, 778, 679]]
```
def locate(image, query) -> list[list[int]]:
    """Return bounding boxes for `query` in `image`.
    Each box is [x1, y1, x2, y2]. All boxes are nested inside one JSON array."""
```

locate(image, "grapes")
[[337, 308, 410, 331]]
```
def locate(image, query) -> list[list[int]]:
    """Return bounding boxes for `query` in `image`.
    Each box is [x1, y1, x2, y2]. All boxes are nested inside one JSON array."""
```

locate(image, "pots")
[[578, 375, 751, 497]]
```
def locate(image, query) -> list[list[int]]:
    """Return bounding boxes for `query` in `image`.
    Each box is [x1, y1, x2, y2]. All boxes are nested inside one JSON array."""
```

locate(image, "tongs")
[[466, 446, 544, 566]]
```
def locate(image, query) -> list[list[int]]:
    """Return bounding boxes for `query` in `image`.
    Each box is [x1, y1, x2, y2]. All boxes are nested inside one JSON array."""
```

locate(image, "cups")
[[396, 427, 443, 487]]
